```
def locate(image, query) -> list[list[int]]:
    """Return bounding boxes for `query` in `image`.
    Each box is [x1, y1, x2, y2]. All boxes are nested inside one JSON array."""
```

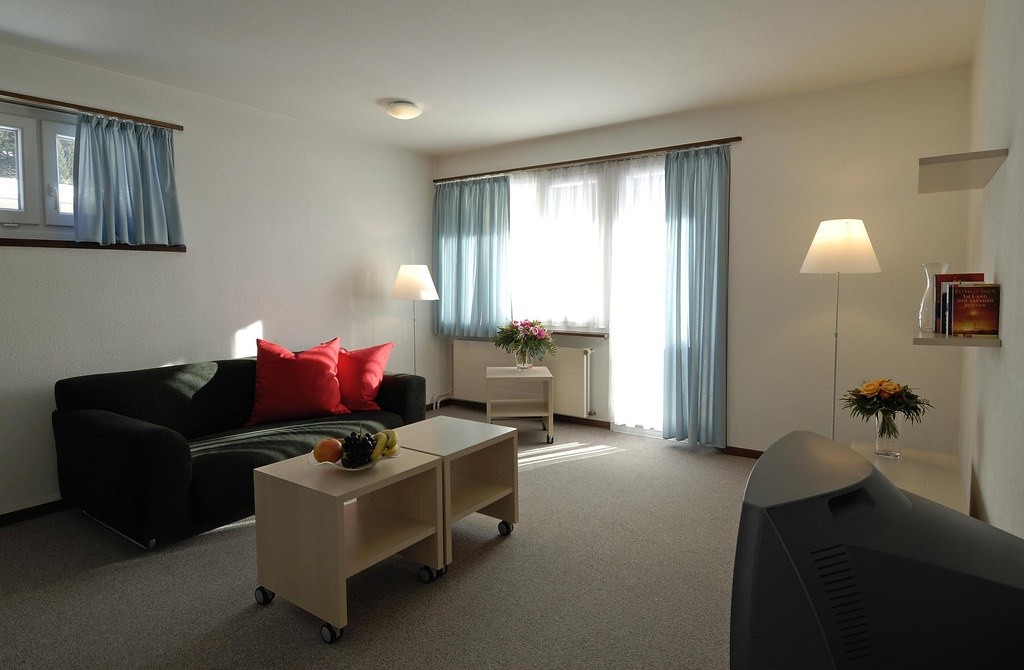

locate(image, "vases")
[[918, 262, 949, 331], [875, 408, 901, 459], [515, 346, 532, 373]]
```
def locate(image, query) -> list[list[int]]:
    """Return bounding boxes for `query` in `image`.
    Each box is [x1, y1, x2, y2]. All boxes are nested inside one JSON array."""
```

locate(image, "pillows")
[[249, 338, 351, 426], [322, 341, 397, 412]]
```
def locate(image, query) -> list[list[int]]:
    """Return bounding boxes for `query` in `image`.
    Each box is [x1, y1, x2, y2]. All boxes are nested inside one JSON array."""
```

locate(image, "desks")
[[850, 440, 973, 516]]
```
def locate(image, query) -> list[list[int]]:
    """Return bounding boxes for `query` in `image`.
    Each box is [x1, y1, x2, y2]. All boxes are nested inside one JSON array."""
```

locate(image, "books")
[[932, 272, 1001, 338]]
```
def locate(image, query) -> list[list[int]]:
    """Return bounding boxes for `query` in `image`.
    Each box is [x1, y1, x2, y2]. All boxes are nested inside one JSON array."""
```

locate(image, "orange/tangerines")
[[314, 438, 343, 463]]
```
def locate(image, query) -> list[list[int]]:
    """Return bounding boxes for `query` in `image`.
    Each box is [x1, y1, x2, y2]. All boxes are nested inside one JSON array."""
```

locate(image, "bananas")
[[370, 430, 398, 461]]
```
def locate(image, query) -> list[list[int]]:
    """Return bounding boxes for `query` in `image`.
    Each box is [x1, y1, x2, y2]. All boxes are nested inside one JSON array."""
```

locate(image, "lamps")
[[385, 102, 422, 120], [392, 265, 440, 375], [799, 218, 882, 442]]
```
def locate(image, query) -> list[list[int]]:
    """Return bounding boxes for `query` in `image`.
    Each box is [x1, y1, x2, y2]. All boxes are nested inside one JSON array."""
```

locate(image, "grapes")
[[341, 431, 377, 468]]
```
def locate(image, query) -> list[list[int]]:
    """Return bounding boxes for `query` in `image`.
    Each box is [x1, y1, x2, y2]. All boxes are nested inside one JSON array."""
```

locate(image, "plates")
[[309, 448, 402, 471]]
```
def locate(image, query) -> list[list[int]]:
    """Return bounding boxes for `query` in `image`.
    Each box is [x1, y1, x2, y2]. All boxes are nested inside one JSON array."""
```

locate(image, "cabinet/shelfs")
[[393, 415, 520, 574], [253, 447, 445, 643], [486, 366, 555, 443]]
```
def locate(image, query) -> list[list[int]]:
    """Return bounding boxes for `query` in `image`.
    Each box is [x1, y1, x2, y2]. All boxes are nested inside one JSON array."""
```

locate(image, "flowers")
[[838, 378, 935, 440], [488, 317, 558, 363]]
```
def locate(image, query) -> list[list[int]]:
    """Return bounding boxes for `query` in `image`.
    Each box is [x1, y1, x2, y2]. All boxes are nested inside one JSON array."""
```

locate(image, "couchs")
[[51, 350, 427, 551]]
